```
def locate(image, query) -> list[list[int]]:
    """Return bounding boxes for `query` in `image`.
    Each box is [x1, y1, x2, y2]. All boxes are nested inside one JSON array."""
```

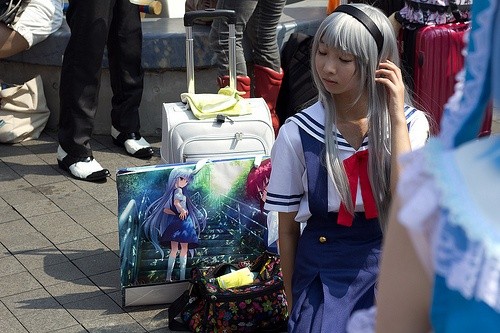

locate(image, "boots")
[[216, 75, 251, 99], [253, 64, 284, 138]]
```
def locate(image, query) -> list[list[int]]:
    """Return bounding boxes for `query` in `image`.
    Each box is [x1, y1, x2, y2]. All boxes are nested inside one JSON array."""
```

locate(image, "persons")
[[57, 0, 154, 181], [263, 4, 439, 333], [0, 0, 63, 60], [208, 0, 284, 137], [367, 0, 500, 333]]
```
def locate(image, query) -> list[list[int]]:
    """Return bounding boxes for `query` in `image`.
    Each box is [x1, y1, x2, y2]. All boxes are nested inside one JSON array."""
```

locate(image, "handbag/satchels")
[[0, 0, 30, 26], [167, 251, 289, 333], [0, 74, 50, 143], [116, 154, 279, 307]]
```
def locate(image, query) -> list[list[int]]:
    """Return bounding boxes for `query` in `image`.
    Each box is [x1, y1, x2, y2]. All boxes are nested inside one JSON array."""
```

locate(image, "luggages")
[[161, 8, 276, 177], [413, 20, 493, 138]]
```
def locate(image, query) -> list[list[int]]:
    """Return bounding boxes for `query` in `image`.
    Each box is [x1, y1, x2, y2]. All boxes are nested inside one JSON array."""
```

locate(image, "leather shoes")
[[56, 145, 111, 180], [110, 125, 154, 161]]
